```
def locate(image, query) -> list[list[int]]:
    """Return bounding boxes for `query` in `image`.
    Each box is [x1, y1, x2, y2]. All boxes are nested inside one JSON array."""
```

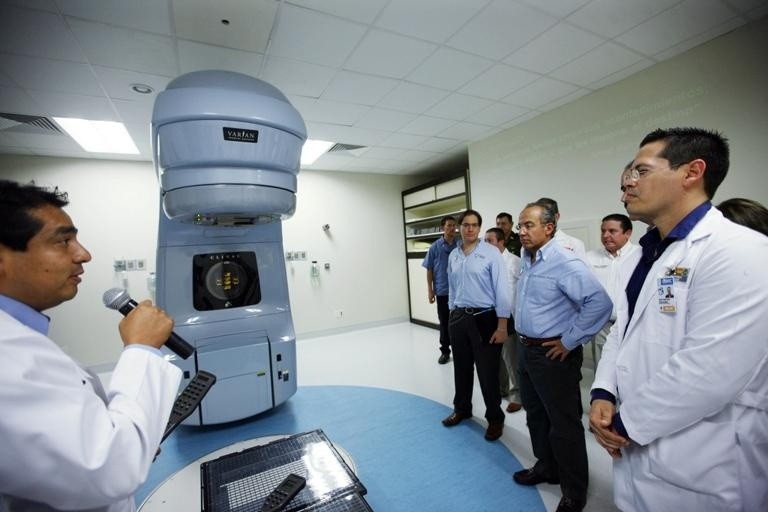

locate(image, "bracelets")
[[497, 328, 507, 332]]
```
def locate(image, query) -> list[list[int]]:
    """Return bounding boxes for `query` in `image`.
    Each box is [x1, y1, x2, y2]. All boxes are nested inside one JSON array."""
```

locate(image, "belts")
[[458, 307, 488, 314], [517, 332, 554, 346]]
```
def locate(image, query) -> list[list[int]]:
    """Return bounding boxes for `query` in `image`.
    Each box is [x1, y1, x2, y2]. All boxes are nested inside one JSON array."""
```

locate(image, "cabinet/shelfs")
[[403, 176, 467, 327]]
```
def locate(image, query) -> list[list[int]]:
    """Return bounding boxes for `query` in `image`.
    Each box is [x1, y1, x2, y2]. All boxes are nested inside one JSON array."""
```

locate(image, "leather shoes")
[[442, 412, 472, 426], [556, 495, 586, 512], [506, 403, 521, 412], [514, 469, 561, 485], [486, 423, 502, 440], [439, 354, 449, 364]]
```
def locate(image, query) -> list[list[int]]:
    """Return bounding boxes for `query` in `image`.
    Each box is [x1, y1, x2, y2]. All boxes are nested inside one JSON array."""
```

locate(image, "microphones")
[[103, 287, 197, 360]]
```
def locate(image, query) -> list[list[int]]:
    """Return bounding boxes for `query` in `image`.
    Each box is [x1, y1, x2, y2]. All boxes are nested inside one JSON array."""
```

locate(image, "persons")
[[422, 216, 458, 363], [484, 227, 523, 412], [587, 128, 768, 511], [536, 197, 585, 260], [512, 203, 613, 509], [588, 213, 643, 434], [716, 197, 768, 233], [496, 212, 520, 258], [0, 175, 184, 511], [441, 210, 505, 441]]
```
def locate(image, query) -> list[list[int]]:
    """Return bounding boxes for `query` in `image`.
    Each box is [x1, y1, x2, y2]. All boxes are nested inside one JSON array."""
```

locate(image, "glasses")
[[623, 164, 669, 181]]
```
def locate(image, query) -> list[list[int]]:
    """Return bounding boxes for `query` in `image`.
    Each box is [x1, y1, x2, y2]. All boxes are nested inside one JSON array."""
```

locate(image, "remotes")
[[260, 474, 306, 512], [159, 369, 219, 445]]
